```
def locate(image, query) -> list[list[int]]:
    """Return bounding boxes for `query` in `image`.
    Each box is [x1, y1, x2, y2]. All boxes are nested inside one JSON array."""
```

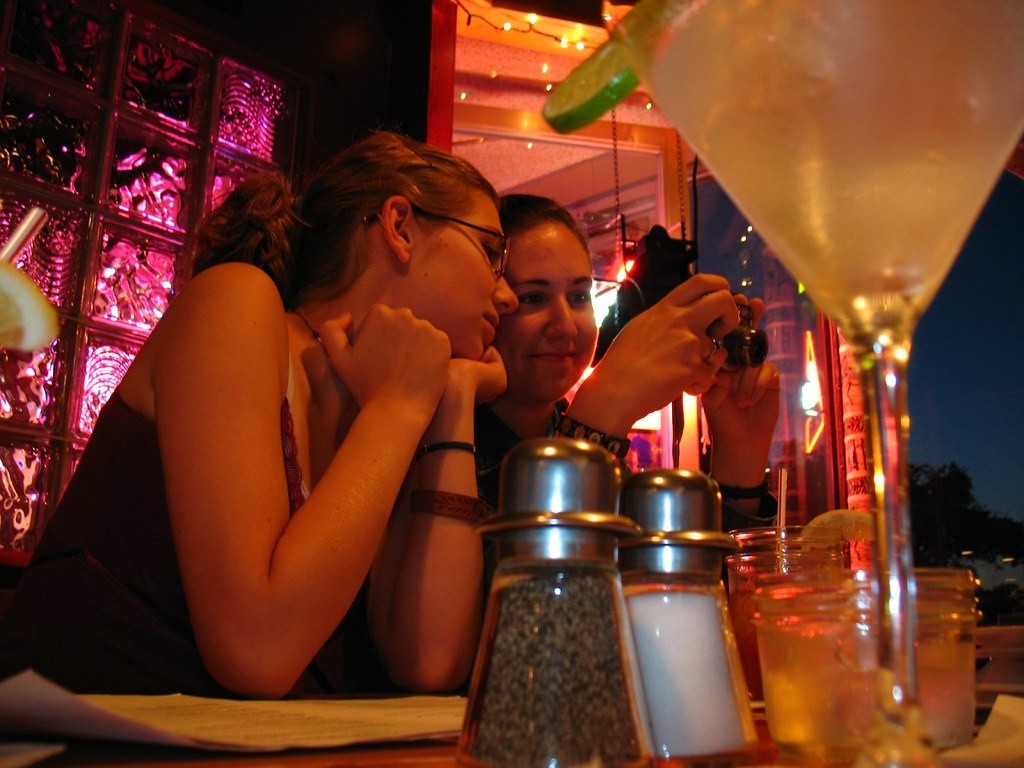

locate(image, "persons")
[[0, 130, 507, 702], [473, 193, 779, 594]]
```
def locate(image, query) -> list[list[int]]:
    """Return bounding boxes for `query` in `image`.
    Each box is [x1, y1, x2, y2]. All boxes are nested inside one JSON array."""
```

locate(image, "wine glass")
[[612, 0, 1024, 768]]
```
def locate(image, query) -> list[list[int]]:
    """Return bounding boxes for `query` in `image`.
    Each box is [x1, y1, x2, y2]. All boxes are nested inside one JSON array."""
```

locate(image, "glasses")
[[363, 205, 510, 276]]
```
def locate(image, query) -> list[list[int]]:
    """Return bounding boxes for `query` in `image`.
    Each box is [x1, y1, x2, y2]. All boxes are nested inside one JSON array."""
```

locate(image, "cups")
[[914, 568, 978, 749], [726, 526, 844, 711], [752, 568, 883, 768]]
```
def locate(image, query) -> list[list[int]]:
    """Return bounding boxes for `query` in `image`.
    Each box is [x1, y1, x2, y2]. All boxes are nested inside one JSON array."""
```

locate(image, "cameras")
[[618, 225, 767, 370]]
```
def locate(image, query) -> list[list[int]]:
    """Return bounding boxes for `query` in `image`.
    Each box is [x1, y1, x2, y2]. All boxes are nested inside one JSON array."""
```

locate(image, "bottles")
[[616, 467, 760, 768], [455, 435, 650, 768]]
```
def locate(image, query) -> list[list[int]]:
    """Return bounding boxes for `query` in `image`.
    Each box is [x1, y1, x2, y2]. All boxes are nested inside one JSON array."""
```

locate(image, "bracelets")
[[407, 488, 487, 524], [722, 475, 771, 501], [415, 442, 477, 458], [550, 399, 632, 459]]
